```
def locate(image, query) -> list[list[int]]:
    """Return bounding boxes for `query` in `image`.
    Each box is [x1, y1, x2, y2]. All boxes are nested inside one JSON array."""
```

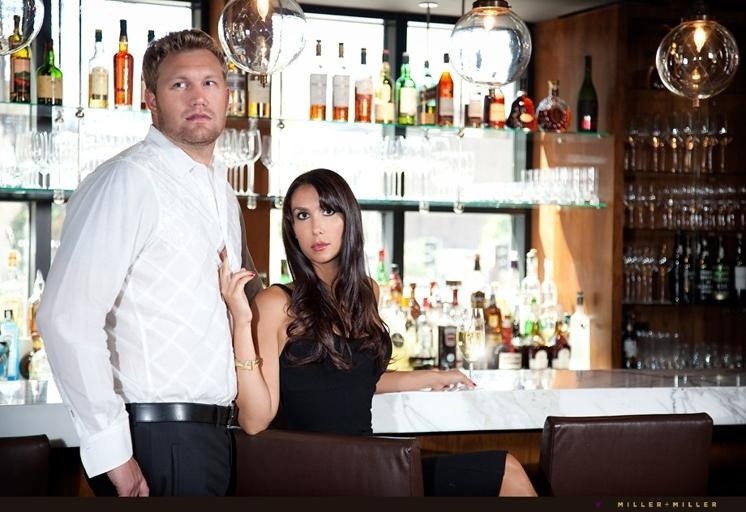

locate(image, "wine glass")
[[624, 238, 671, 303], [626, 109, 739, 177], [1, 132, 137, 188], [627, 181, 738, 229], [215, 128, 262, 196], [456, 307, 489, 387]]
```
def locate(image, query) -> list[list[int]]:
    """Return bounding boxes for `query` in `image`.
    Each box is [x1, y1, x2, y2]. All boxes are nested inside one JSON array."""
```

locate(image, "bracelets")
[[233, 357, 263, 370]]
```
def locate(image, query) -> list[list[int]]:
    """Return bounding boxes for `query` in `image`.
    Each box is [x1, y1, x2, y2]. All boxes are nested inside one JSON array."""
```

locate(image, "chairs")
[[539, 414, 715, 497], [228, 425, 424, 499], [1, 434, 65, 496]]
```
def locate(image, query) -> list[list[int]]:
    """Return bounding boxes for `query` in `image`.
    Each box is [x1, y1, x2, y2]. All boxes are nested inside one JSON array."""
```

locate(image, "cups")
[[520, 165, 598, 205], [636, 330, 743, 371]]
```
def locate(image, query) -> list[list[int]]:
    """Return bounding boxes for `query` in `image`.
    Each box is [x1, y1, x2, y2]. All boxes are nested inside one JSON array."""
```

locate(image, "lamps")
[[447, 0, 532, 101], [217, 1, 308, 88], [0, 1, 46, 57], [654, 1, 741, 108]]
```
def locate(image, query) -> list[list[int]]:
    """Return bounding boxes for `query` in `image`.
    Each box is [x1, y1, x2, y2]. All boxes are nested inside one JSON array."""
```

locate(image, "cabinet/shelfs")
[[213, 1, 612, 370], [526, 0, 745, 369], [0, 0, 212, 381]]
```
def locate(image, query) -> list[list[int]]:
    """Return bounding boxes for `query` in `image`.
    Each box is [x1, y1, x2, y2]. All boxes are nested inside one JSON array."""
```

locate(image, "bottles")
[[309, 38, 327, 119], [249, 34, 272, 118], [374, 50, 394, 124], [622, 310, 639, 369], [526, 336, 549, 369], [395, 52, 415, 125], [490, 244, 511, 283], [525, 296, 541, 336], [36, 41, 64, 108], [569, 290, 593, 371], [439, 53, 454, 127], [333, 40, 351, 120], [623, 239, 670, 300], [696, 233, 714, 302], [387, 263, 401, 295], [8, 13, 35, 100], [490, 83, 506, 128], [470, 292, 488, 369], [717, 115, 733, 172], [4, 250, 26, 310], [521, 252, 541, 294], [114, 19, 136, 106], [511, 322, 529, 370], [548, 322, 570, 369], [729, 232, 746, 307], [540, 258, 557, 320], [354, 47, 373, 121], [89, 28, 107, 106], [501, 310, 514, 343], [445, 280, 467, 368], [376, 247, 390, 288], [510, 251, 523, 291], [484, 295, 501, 333], [3, 313, 20, 379], [682, 232, 699, 303], [626, 183, 745, 232], [463, 78, 485, 126], [418, 59, 436, 125], [512, 78, 537, 128], [425, 282, 442, 369], [468, 251, 486, 289], [226, 55, 248, 117], [672, 231, 686, 304], [712, 237, 730, 302], [27, 268, 53, 351], [539, 80, 571, 130], [141, 29, 154, 108], [625, 116, 715, 173], [407, 283, 422, 320]]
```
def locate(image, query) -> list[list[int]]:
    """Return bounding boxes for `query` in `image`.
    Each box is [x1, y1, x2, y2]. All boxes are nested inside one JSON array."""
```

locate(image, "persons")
[[218, 168, 538, 498], [36, 29, 244, 498]]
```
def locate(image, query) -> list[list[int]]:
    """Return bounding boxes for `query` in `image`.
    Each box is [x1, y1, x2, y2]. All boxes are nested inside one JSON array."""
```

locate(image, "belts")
[[125, 402, 237, 427]]
